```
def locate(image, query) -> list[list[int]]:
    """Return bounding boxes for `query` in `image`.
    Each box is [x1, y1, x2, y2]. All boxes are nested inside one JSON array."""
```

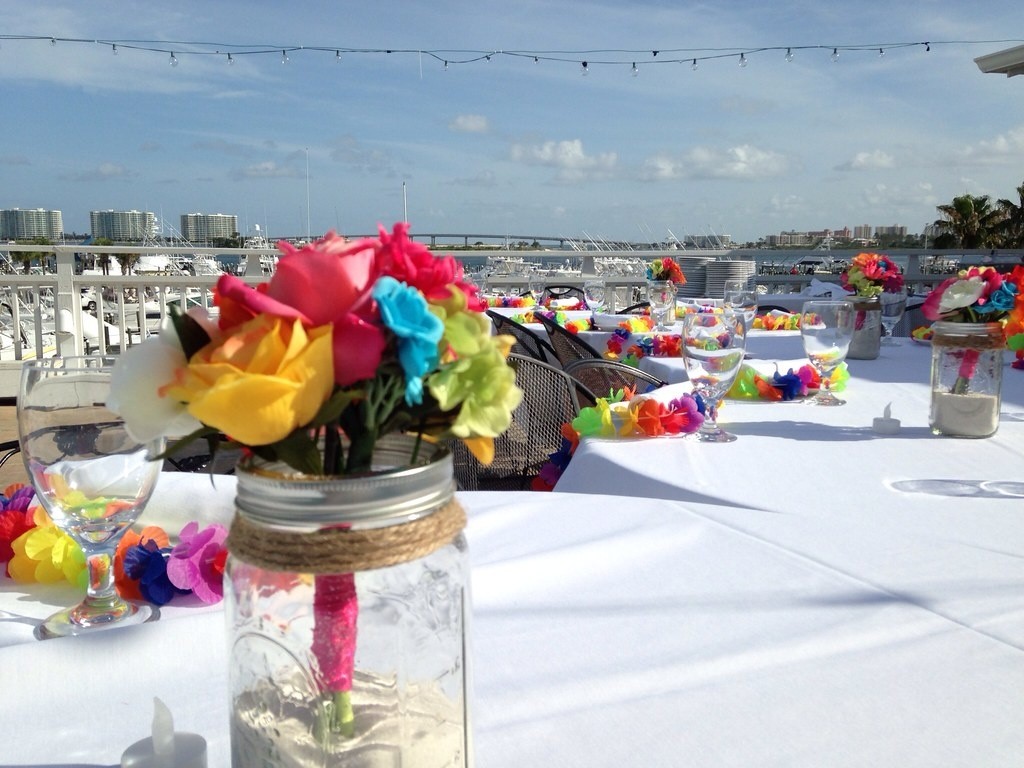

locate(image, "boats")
[[1, 213, 281, 360], [464, 255, 658, 310]]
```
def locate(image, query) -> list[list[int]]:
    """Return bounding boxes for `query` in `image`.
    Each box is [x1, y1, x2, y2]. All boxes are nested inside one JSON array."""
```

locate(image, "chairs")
[[413, 352, 605, 493], [484, 309, 562, 374], [533, 311, 642, 401], [518, 284, 591, 309], [562, 358, 668, 421], [617, 302, 653, 314]]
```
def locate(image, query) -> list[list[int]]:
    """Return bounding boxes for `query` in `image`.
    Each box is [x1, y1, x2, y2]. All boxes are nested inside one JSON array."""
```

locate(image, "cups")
[[722, 280, 747, 292]]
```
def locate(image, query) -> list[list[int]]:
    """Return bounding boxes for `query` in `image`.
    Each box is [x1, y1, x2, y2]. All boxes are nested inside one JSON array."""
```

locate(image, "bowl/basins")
[[594, 314, 638, 325]]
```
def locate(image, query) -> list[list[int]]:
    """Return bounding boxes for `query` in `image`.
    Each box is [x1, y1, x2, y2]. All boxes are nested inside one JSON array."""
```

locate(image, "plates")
[[594, 325, 620, 332], [913, 336, 932, 346], [675, 256, 757, 299]]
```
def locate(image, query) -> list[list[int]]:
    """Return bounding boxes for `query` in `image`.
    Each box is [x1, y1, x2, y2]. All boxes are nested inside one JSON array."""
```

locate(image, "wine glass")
[[583, 279, 606, 316], [470, 264, 487, 303], [723, 292, 759, 355], [648, 279, 676, 332], [879, 285, 908, 346], [800, 301, 855, 406], [15, 355, 162, 642], [528, 274, 545, 310], [681, 312, 747, 443]]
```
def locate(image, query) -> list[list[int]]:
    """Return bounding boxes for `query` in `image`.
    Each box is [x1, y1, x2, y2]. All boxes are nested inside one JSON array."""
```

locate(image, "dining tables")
[[550, 280, 1024, 553], [0, 464, 1024, 768]]
